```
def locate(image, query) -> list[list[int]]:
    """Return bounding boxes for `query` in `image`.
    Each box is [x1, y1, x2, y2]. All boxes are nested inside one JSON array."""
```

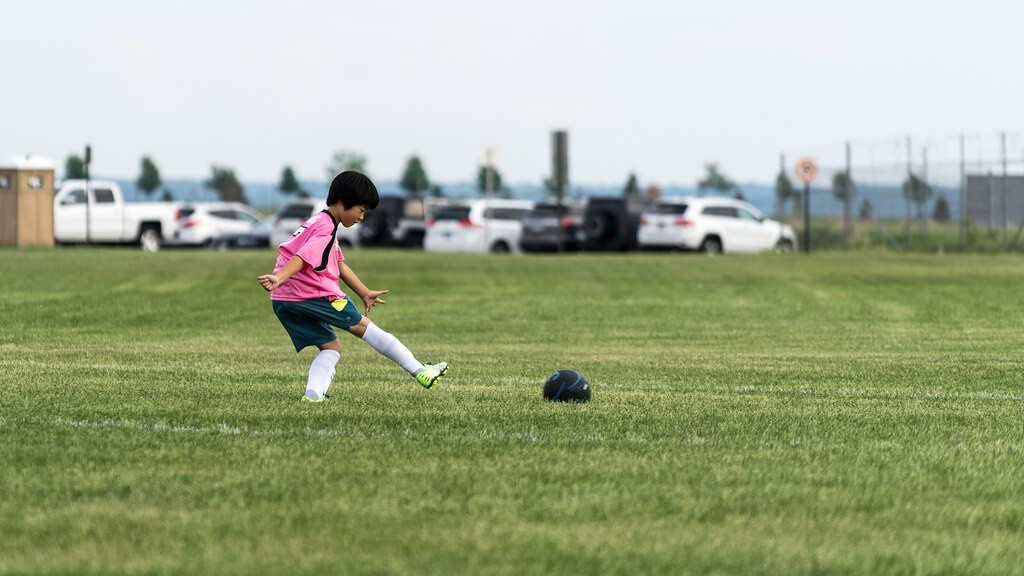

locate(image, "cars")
[[266, 198, 367, 249], [178, 200, 271, 251], [637, 196, 801, 259], [357, 195, 452, 248], [423, 200, 538, 256], [517, 195, 644, 251]]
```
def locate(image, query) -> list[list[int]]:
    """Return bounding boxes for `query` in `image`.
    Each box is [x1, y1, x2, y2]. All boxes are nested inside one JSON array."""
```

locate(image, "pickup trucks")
[[49, 177, 185, 254]]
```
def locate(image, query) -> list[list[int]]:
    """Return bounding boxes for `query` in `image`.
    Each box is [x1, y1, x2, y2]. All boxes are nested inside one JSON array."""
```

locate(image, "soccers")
[[545, 369, 592, 404]]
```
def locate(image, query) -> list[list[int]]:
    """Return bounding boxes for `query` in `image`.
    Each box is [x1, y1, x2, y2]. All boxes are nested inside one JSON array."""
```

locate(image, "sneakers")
[[300, 395, 328, 403], [416, 360, 449, 390]]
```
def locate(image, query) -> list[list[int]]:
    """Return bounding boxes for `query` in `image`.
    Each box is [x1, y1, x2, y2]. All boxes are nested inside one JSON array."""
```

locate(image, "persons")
[[257, 170, 450, 404]]
